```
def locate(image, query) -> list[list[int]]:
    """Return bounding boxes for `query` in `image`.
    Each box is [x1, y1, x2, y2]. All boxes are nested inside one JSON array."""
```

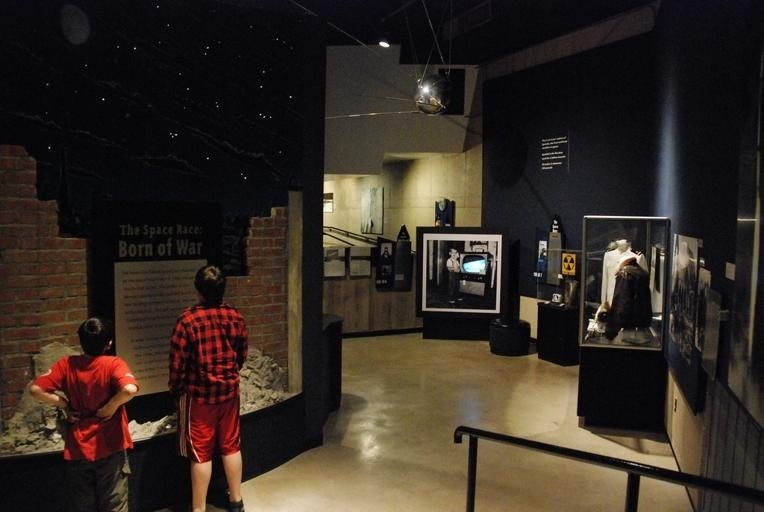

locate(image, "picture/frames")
[[416, 226, 508, 320]]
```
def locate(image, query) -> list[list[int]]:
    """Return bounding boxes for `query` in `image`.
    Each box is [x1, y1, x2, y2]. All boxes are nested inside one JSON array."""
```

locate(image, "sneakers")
[[222, 486, 245, 512]]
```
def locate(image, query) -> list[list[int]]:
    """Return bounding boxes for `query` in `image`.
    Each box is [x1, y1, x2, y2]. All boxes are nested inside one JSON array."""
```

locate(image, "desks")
[[537, 301, 593, 367]]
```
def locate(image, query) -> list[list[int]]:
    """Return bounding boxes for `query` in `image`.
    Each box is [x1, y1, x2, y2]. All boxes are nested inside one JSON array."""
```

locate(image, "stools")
[[490, 318, 530, 356]]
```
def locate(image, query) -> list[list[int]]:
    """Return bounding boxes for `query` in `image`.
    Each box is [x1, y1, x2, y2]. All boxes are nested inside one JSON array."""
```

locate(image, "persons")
[[602, 256, 654, 344], [443, 248, 465, 304], [28, 316, 140, 512], [167, 263, 249, 511], [599, 238, 649, 311]]
